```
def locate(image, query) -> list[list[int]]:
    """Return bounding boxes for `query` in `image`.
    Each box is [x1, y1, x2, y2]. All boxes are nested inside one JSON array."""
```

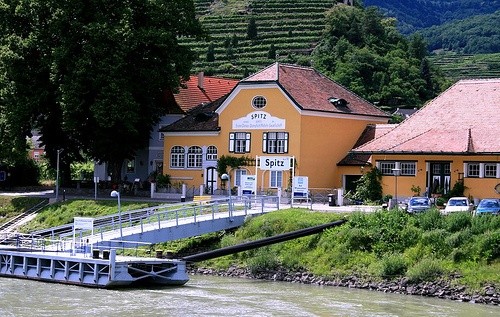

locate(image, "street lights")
[[392, 169, 401, 211], [110, 191, 123, 243], [221, 174, 233, 221]]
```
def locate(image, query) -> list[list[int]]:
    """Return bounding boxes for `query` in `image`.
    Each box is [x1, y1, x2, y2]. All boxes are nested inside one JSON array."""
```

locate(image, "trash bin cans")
[[327, 194, 336, 206]]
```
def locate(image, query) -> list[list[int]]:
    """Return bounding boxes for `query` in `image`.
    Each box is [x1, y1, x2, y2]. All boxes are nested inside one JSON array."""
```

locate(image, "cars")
[[443, 197, 473, 218], [404, 197, 432, 213], [475, 199, 500, 216]]
